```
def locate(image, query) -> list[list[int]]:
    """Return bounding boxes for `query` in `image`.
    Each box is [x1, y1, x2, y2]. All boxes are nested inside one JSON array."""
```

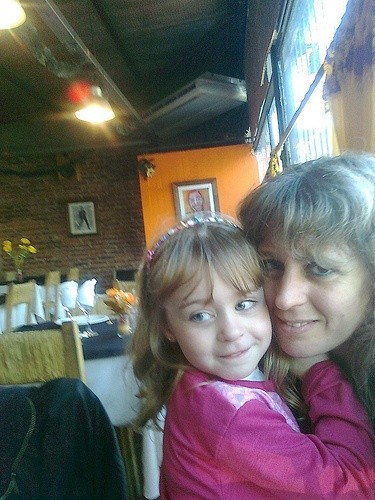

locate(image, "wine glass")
[[58, 287, 77, 320], [76, 285, 98, 337]]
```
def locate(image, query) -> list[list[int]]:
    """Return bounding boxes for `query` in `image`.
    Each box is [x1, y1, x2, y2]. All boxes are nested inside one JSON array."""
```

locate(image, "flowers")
[[2, 237, 38, 269], [103, 288, 136, 326]]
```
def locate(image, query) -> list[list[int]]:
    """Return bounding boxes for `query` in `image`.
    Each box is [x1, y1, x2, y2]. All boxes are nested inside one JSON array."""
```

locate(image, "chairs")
[[0, 320, 85, 385], [0, 269, 15, 284], [66, 267, 80, 283], [5, 279, 34, 330], [43, 270, 60, 321]]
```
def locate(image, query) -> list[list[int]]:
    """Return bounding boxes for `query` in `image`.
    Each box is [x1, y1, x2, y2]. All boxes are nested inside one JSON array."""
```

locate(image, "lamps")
[[0, 0, 26, 29], [74, 85, 114, 124]]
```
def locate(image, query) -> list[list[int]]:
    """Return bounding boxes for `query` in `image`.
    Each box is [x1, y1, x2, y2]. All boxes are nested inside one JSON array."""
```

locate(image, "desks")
[[13, 317, 148, 500]]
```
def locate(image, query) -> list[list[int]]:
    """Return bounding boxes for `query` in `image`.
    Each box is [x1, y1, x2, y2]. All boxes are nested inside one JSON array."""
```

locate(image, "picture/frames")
[[67, 199, 99, 237], [169, 177, 221, 221]]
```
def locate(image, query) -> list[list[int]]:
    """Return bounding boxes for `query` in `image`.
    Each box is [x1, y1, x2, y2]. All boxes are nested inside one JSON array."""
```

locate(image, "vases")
[[16, 268, 23, 283], [116, 312, 131, 339]]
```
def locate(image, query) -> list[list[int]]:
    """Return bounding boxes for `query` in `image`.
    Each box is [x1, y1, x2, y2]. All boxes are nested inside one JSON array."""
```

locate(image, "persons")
[[122, 210, 375, 500], [235, 154, 375, 432], [78, 206, 91, 231]]
